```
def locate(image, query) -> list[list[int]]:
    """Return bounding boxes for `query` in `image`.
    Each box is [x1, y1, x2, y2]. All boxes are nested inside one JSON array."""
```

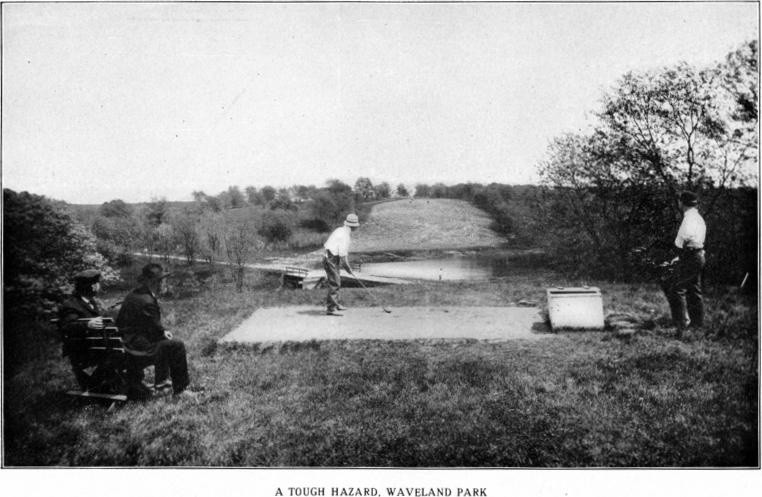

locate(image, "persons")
[[319, 213, 363, 318], [656, 189, 711, 338], [114, 262, 208, 400], [55, 268, 123, 377]]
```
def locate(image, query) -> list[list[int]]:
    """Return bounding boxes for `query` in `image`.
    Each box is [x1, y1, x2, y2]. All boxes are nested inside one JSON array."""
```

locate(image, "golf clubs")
[[352, 274, 392, 313]]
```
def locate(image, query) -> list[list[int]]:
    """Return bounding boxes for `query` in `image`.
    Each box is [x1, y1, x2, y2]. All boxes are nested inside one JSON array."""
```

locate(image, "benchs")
[[50, 316, 148, 413]]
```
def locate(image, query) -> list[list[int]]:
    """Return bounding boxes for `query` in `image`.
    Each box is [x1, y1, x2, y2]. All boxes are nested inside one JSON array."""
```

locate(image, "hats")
[[680, 192, 698, 205], [344, 214, 360, 227], [136, 263, 172, 285], [74, 270, 105, 284]]
